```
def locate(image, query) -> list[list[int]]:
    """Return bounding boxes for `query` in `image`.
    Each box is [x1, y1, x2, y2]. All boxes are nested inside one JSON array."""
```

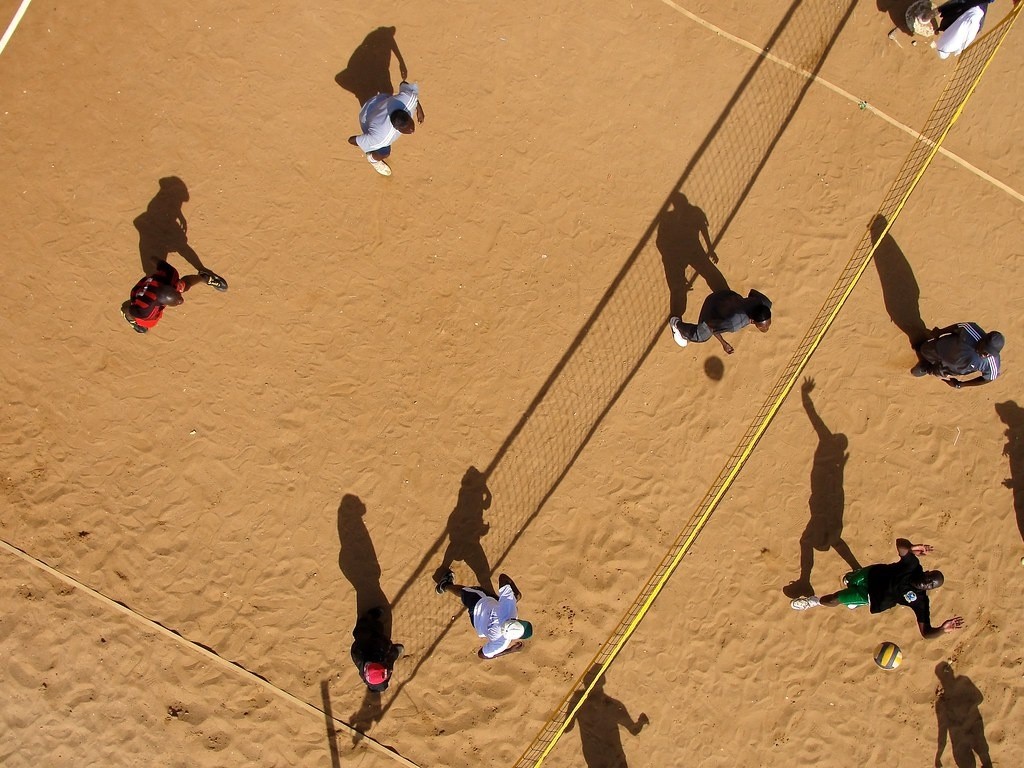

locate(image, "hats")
[[981, 331, 1005, 356], [390, 109, 415, 134], [363, 662, 388, 685], [501, 620, 532, 640], [746, 301, 771, 322]]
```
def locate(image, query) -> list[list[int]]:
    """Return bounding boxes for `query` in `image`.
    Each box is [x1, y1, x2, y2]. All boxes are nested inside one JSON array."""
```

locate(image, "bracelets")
[[956, 381, 961, 389]]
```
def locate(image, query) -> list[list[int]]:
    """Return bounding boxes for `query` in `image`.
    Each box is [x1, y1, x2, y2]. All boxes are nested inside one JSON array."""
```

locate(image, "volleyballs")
[[873, 642, 903, 671]]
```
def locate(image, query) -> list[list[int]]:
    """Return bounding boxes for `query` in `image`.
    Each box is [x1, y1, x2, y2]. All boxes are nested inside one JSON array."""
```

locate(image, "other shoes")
[[394, 645, 404, 654], [363, 608, 384, 619], [911, 364, 931, 376]]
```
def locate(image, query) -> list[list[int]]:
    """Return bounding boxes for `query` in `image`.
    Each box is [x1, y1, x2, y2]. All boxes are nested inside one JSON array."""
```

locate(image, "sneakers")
[[367, 154, 391, 176], [123, 313, 147, 333], [790, 596, 820, 610], [198, 270, 228, 291], [670, 317, 688, 346], [434, 570, 455, 595]]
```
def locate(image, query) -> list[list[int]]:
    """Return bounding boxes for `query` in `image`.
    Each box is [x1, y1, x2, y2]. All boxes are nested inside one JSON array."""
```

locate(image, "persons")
[[790, 538, 965, 640], [669, 290, 773, 354], [434, 569, 533, 660], [911, 321, 1005, 389], [348, 81, 425, 176], [918, 0, 996, 60], [120, 256, 228, 333], [350, 607, 405, 694]]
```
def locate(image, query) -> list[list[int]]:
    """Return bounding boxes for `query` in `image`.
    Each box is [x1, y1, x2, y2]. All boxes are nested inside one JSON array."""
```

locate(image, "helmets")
[[157, 286, 181, 306]]
